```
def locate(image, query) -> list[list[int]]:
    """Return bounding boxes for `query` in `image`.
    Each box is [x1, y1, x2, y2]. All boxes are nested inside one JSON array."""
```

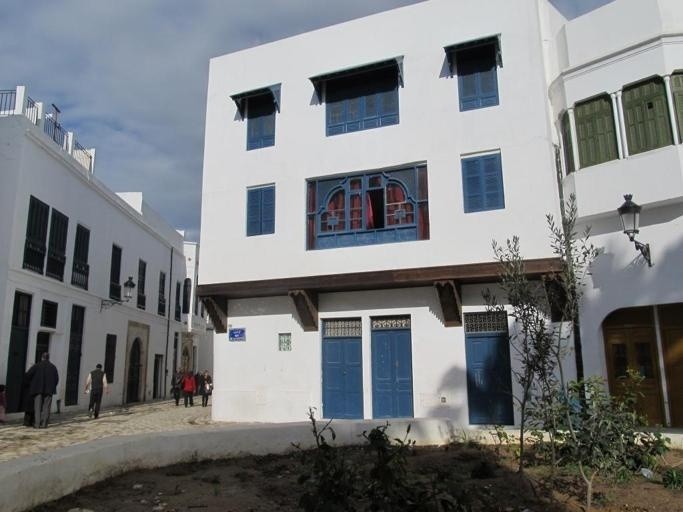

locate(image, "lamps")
[[96, 275, 137, 314], [614, 192, 656, 270]]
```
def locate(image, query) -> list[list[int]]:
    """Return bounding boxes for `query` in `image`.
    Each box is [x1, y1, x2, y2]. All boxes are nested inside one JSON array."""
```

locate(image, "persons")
[[28, 352, 58, 428], [0, 384, 7, 422], [19, 364, 35, 426], [170, 369, 212, 407], [81, 363, 110, 418]]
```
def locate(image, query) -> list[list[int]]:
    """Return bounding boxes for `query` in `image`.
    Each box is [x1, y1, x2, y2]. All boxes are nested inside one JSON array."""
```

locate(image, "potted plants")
[[436, 423, 483, 481]]
